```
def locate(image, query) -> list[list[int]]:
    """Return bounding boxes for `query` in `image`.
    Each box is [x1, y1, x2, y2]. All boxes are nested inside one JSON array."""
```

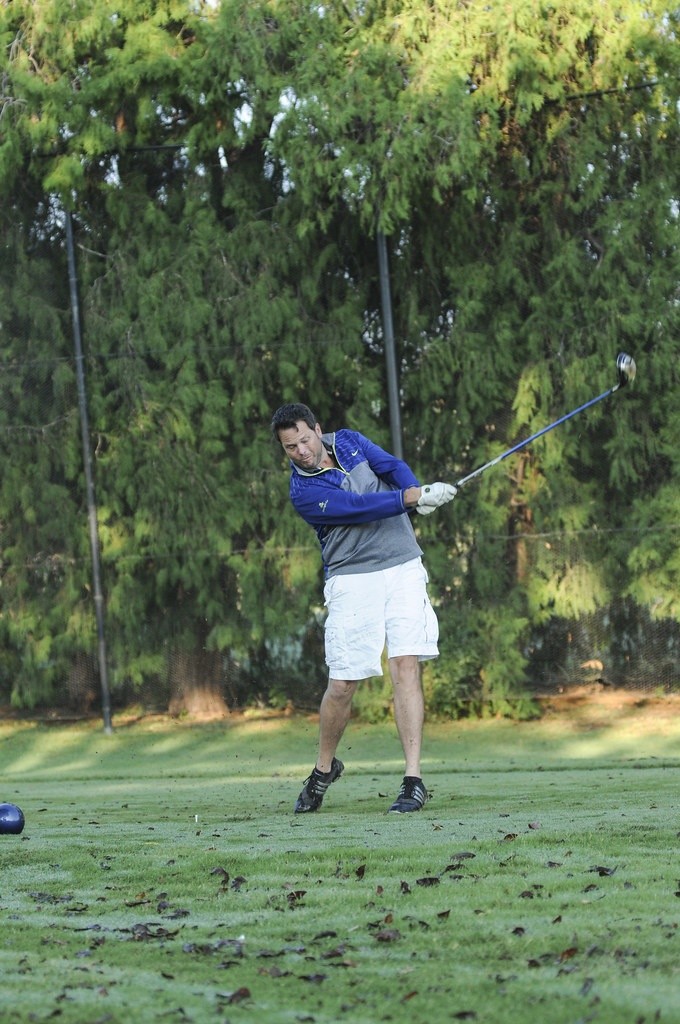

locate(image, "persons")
[[271, 402, 458, 814]]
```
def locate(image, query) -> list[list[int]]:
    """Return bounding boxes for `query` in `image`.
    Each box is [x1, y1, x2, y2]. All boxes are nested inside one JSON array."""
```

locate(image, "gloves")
[[418, 482, 458, 505], [416, 504, 437, 515]]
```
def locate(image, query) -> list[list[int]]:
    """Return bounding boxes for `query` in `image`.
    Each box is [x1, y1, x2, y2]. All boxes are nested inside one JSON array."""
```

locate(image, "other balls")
[[0, 802, 26, 835]]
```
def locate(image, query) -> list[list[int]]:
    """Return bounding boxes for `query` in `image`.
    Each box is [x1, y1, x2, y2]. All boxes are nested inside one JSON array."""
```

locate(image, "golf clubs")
[[453, 352, 637, 489]]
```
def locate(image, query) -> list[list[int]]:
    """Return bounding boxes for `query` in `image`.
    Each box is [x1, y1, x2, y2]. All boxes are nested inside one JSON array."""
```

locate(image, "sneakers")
[[389, 776, 427, 813], [294, 757, 345, 814]]
